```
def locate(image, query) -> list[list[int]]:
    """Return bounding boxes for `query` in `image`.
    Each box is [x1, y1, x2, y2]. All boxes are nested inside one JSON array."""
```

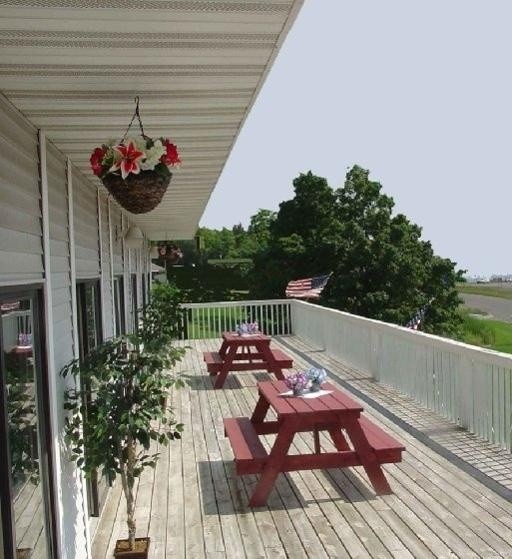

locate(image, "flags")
[[1, 302, 20, 311], [406, 301, 431, 330], [285, 274, 330, 299], [19, 333, 32, 346]]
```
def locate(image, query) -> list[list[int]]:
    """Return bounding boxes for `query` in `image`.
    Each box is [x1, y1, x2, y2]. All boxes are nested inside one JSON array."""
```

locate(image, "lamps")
[[151, 247, 159, 258], [125, 226, 144, 248]]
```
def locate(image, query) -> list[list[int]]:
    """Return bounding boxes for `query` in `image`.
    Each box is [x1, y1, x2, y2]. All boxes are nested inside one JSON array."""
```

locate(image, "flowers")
[[303, 367, 327, 384], [90, 137, 181, 180], [285, 371, 308, 390], [158, 241, 183, 258]]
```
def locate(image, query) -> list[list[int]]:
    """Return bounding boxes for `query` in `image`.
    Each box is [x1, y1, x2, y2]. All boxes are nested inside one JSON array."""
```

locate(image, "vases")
[[311, 382, 320, 391], [293, 389, 303, 395]]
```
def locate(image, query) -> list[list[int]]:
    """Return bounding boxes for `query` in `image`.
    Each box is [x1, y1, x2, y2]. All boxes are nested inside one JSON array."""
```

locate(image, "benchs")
[[357, 413, 406, 464], [270, 349, 293, 369], [203, 352, 222, 371], [223, 416, 267, 476]]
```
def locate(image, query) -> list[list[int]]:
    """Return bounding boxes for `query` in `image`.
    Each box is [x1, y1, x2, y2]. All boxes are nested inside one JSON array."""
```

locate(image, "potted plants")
[[127, 279, 193, 414], [5, 350, 39, 559], [59, 336, 185, 559]]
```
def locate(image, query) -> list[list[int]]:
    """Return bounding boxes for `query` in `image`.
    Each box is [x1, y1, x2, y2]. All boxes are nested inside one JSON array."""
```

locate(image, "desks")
[[210, 331, 285, 389], [248, 379, 392, 506]]
